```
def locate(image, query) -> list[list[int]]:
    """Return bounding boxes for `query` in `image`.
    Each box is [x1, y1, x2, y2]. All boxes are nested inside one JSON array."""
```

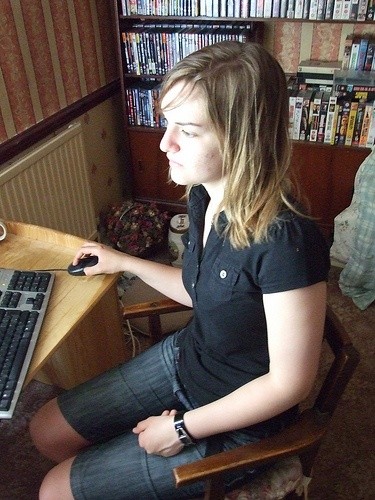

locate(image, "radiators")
[[0, 123, 97, 240]]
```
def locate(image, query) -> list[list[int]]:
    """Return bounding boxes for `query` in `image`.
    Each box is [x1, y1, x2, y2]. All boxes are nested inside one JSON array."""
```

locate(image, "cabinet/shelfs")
[[113, 0, 375, 227]]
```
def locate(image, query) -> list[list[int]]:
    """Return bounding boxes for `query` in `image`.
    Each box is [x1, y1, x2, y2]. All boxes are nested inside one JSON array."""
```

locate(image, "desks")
[[0, 220, 126, 390]]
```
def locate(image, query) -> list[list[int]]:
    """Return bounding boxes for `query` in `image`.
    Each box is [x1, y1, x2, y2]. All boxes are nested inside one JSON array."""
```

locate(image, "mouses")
[[68, 255, 103, 275]]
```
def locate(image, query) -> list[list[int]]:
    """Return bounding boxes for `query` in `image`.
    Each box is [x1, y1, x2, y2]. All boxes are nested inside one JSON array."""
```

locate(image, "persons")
[[29, 41, 327, 500]]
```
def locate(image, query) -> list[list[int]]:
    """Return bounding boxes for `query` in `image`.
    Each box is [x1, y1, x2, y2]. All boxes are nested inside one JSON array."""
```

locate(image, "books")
[[120, 0, 375, 22], [282, 36, 375, 149], [125, 84, 167, 128], [124, 23, 250, 75]]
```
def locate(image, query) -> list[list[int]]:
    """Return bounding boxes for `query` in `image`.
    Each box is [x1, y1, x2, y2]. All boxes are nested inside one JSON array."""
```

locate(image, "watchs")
[[174, 411, 195, 447]]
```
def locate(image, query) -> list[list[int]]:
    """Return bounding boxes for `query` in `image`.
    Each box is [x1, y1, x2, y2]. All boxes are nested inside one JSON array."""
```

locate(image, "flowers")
[[99, 201, 171, 254]]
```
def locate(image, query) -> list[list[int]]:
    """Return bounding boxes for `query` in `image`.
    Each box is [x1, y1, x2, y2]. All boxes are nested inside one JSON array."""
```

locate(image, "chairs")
[[123, 300, 361, 500]]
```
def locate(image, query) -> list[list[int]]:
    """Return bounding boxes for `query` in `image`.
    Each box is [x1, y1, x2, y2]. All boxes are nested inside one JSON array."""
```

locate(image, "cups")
[[0, 221, 7, 242]]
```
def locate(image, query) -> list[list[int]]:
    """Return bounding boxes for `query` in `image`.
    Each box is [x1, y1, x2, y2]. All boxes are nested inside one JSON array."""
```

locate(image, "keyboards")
[[0, 267, 56, 419]]
